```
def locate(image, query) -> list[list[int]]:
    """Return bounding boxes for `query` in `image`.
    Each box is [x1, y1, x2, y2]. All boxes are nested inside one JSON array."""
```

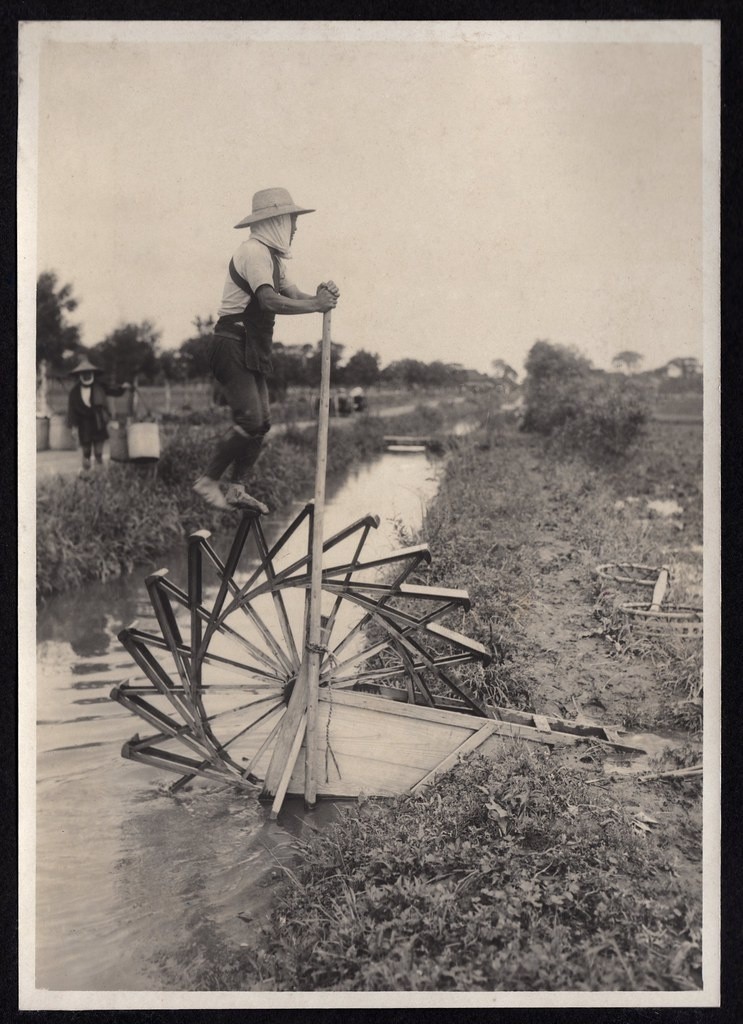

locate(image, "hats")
[[70, 359, 102, 376], [233, 187, 316, 228]]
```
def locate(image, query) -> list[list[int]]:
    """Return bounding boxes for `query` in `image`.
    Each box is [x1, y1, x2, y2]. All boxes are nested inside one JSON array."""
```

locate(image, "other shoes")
[[194, 478, 237, 514], [225, 482, 270, 516]]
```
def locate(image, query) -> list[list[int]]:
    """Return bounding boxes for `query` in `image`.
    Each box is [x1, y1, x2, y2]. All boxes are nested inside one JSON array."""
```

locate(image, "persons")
[[191, 187, 340, 515], [65, 360, 130, 471]]
